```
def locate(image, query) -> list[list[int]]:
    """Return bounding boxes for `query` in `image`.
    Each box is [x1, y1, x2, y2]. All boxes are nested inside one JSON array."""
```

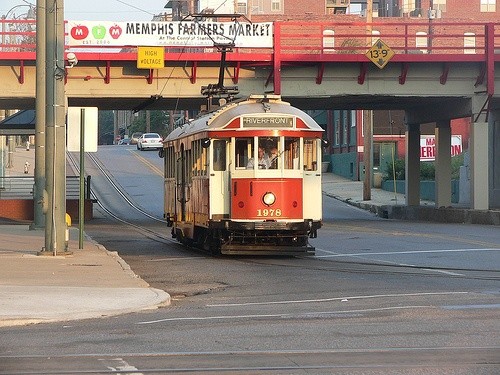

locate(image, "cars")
[[136, 132, 163, 151], [118, 139, 131, 145]]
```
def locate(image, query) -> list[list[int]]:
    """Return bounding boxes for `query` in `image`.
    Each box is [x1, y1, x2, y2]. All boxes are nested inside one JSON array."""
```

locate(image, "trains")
[[158, 95, 326, 259]]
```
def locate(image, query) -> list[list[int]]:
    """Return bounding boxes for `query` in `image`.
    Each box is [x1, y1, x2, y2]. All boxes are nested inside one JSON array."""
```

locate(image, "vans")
[[130, 132, 143, 145]]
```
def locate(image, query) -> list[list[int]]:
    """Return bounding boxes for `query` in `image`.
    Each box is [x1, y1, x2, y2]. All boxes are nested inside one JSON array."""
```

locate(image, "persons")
[[246, 147, 272, 169]]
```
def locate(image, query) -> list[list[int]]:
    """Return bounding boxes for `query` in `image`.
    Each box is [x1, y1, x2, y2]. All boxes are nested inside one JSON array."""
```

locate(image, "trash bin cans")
[[373, 173, 382, 185]]
